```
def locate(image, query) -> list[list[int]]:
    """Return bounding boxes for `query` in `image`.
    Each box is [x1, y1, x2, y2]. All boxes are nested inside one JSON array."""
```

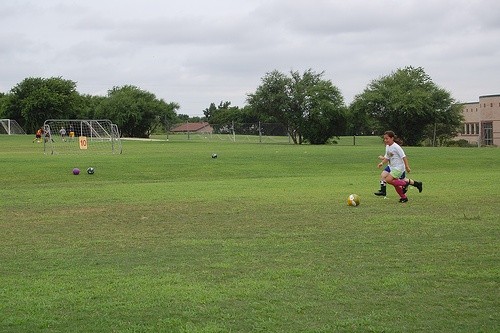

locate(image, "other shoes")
[[402, 185, 407, 193], [399, 197, 408, 202]]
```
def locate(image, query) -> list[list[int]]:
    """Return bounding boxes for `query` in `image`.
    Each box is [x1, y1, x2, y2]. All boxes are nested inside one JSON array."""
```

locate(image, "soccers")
[[72, 168, 80, 175], [347, 193, 361, 207], [212, 153, 218, 158], [87, 167, 95, 174]]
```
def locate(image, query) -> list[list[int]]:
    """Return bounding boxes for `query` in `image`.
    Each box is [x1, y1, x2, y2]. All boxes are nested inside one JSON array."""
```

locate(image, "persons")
[[44, 126, 49, 143], [374, 131, 422, 202], [60, 126, 66, 142], [32, 128, 43, 144], [69, 124, 75, 142]]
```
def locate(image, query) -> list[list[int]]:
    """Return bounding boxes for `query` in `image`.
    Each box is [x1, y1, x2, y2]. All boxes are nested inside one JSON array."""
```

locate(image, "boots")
[[412, 181, 422, 193], [374, 184, 386, 196]]
